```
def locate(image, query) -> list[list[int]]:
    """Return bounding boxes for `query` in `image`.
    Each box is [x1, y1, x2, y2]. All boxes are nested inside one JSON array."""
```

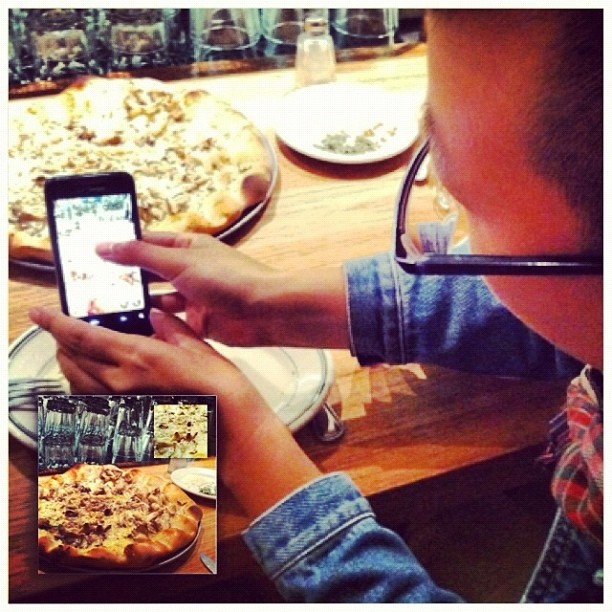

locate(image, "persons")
[[29, 8, 603, 604]]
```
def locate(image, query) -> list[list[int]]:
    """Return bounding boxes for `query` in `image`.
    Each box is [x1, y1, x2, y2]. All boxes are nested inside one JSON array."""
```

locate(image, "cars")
[[111, 396, 155, 468], [259, 8, 337, 61], [189, 8, 260, 76], [39, 394, 76, 471], [89, 8, 189, 74], [78, 393, 117, 464], [10, 12, 86, 80], [330, 6, 399, 69]]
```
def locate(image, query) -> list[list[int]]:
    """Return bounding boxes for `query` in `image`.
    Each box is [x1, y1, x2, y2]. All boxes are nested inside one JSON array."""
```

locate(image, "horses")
[[44, 171, 154, 327]]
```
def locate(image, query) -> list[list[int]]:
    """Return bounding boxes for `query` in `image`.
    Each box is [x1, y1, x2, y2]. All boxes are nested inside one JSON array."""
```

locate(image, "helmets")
[[12, 93, 277, 276], [170, 464, 218, 501], [39, 520, 203, 571], [10, 287, 336, 450], [271, 79, 425, 167]]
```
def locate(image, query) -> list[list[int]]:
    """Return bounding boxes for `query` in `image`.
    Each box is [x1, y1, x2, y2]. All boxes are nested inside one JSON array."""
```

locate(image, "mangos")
[[153, 405, 209, 460], [37, 462, 203, 571], [9, 71, 277, 260]]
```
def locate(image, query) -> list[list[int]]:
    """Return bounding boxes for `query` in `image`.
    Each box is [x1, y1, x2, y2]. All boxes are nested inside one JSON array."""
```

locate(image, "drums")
[[199, 555, 217, 571]]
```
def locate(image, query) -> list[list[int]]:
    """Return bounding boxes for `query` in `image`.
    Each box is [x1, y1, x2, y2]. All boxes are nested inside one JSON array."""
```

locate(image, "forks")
[[10, 378, 74, 410]]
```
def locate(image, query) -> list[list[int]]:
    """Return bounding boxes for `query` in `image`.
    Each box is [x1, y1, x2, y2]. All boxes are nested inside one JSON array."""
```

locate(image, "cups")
[[393, 138, 604, 276]]
